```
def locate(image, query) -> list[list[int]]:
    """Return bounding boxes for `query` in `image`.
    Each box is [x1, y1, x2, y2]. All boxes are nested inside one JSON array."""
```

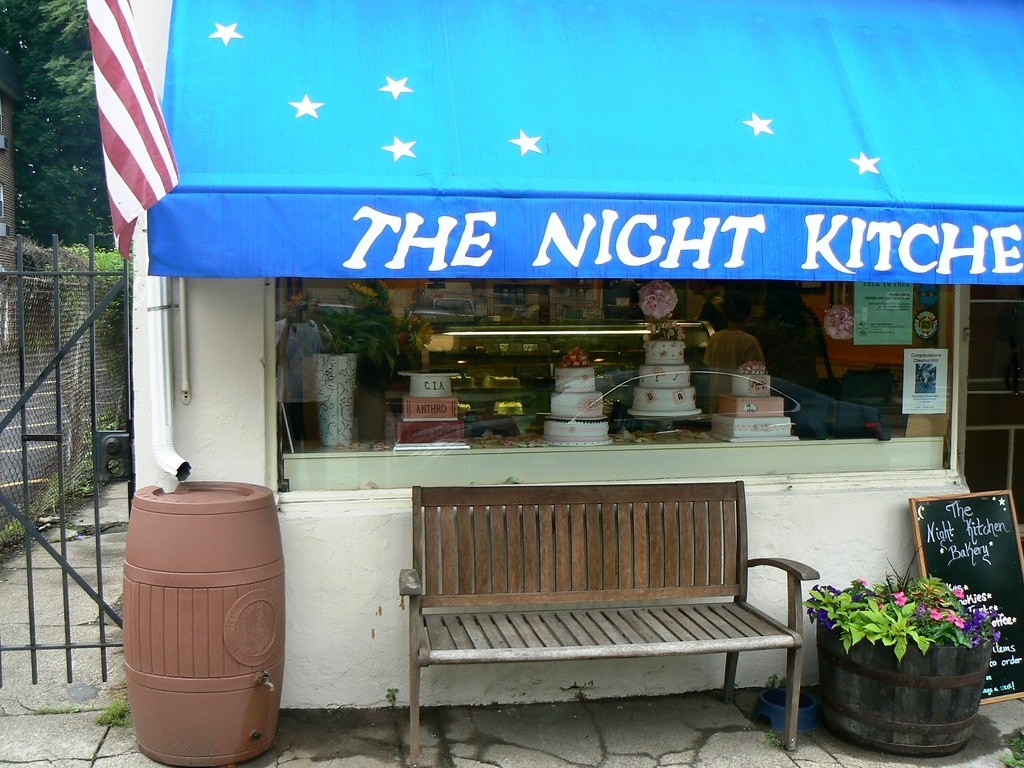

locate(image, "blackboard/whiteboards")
[[910, 490, 1023, 704]]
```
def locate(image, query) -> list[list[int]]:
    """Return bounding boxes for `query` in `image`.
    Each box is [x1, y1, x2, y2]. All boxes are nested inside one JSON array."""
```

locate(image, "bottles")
[[474, 278, 647, 325]]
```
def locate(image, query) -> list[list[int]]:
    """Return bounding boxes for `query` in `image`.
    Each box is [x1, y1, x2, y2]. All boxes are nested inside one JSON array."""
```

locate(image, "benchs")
[[400, 481, 820, 768]]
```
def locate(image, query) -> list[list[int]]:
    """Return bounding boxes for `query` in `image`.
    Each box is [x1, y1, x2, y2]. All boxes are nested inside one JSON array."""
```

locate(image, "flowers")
[[800, 546, 1002, 664], [822, 306, 854, 341], [635, 280, 679, 320], [285, 279, 434, 379]]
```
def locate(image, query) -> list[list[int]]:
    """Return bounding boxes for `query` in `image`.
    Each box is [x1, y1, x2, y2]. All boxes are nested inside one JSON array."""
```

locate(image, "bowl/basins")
[[754, 687, 819, 731]]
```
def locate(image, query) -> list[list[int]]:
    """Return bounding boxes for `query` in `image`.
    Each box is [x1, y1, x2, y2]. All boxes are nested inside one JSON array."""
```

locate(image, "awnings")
[[147, 186, 1024, 285]]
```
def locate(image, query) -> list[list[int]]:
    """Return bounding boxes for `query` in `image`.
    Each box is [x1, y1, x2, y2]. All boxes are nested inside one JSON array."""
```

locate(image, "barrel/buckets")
[[122, 482, 285, 767]]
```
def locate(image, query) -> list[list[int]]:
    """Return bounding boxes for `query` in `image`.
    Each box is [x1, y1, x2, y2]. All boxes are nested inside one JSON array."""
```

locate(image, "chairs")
[[820, 370, 894, 438]]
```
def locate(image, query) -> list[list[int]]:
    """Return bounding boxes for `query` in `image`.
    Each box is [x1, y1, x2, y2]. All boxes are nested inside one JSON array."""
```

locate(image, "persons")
[[703, 282, 818, 415], [274, 291, 333, 453]]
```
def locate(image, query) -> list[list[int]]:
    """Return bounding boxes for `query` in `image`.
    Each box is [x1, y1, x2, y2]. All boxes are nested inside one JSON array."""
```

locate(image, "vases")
[[311, 353, 358, 450], [814, 619, 992, 761]]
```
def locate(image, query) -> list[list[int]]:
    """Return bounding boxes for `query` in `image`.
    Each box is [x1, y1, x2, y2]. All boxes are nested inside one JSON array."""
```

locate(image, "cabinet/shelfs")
[[416, 320, 716, 437]]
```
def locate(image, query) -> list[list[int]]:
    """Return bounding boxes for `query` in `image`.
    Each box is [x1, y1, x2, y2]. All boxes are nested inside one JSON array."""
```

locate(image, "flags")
[[86, 0, 181, 260]]
